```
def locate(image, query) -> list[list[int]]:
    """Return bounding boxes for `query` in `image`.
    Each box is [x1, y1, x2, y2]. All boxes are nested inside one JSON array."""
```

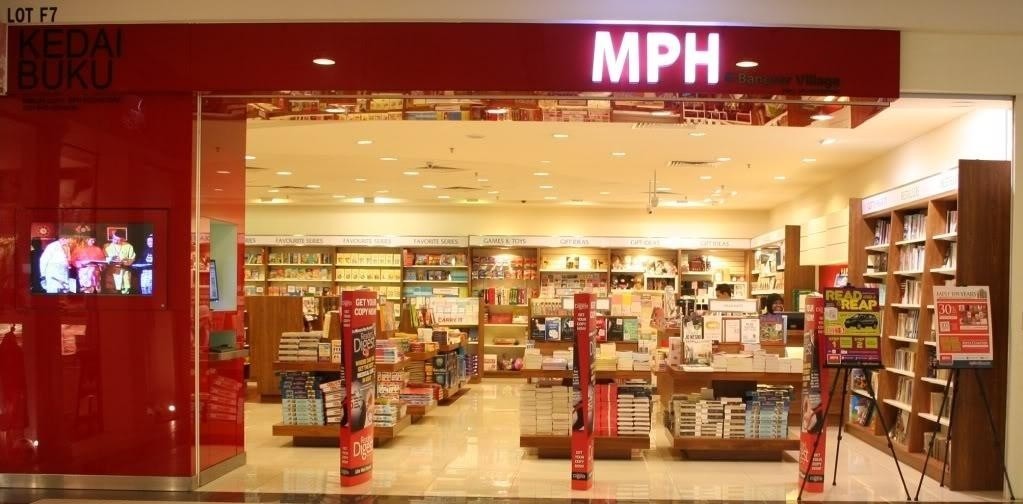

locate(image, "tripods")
[[913, 369, 1016, 501], [796, 368, 911, 501]]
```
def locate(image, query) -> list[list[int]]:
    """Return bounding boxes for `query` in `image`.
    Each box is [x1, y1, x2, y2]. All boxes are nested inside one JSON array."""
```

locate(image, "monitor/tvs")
[[30, 222, 154, 295], [209, 260, 219, 308], [774, 311, 804, 329]]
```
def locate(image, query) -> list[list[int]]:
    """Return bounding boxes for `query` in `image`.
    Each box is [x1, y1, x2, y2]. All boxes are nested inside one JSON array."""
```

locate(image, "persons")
[[30, 232, 136, 295], [128, 232, 155, 295], [711, 279, 735, 305], [762, 292, 790, 341]]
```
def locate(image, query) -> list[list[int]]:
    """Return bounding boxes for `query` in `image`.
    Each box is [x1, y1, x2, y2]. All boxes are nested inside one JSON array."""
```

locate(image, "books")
[[668, 311, 804, 440], [277, 326, 469, 428], [518, 315, 651, 436], [847, 208, 960, 466]]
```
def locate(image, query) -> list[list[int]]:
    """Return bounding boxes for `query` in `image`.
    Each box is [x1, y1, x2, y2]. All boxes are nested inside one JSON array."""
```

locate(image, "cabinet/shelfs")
[[244, 225, 805, 379], [518, 295, 804, 458], [843, 160, 1010, 491], [246, 290, 484, 450]]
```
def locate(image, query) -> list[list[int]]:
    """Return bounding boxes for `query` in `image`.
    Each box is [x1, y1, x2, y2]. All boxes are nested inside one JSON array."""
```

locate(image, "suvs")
[[843, 314, 877, 329]]
